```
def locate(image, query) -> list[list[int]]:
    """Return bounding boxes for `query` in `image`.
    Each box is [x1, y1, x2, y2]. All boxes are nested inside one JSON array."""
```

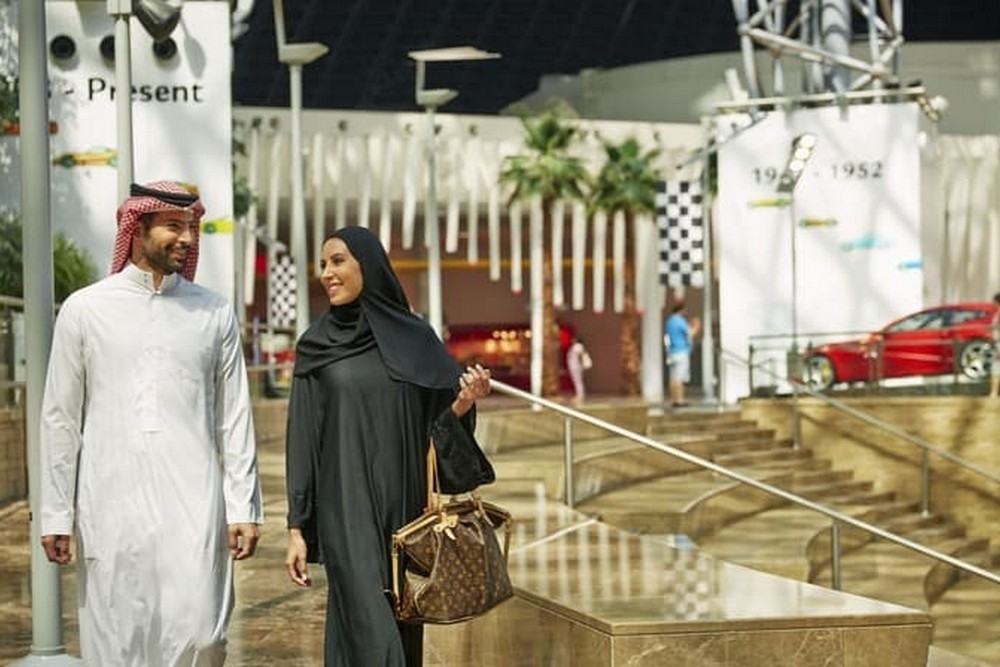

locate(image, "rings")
[[469, 385, 476, 390]]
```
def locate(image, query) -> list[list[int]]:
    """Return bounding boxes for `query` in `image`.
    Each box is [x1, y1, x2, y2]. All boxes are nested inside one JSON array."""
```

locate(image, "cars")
[[799, 302, 1000, 395]]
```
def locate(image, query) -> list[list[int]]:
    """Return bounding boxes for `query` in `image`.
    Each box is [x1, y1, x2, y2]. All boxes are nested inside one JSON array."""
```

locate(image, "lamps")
[[131, 0, 182, 40]]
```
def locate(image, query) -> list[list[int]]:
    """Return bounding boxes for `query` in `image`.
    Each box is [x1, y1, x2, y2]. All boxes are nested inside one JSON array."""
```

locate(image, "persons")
[[284, 225, 491, 667], [664, 304, 702, 407], [39, 181, 265, 667], [986, 291, 999, 399], [551, 317, 593, 404]]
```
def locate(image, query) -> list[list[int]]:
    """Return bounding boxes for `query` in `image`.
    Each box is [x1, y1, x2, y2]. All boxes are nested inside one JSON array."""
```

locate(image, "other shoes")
[[671, 402, 689, 407]]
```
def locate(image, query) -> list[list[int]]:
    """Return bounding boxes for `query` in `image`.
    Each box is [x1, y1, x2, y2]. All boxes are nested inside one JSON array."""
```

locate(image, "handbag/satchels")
[[581, 352, 593, 369], [383, 436, 515, 626]]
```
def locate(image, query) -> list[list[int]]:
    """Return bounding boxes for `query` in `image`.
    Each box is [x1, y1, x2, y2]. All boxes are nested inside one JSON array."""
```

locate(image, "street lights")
[[408, 45, 504, 348], [775, 130, 823, 400], [272, 0, 329, 345]]
